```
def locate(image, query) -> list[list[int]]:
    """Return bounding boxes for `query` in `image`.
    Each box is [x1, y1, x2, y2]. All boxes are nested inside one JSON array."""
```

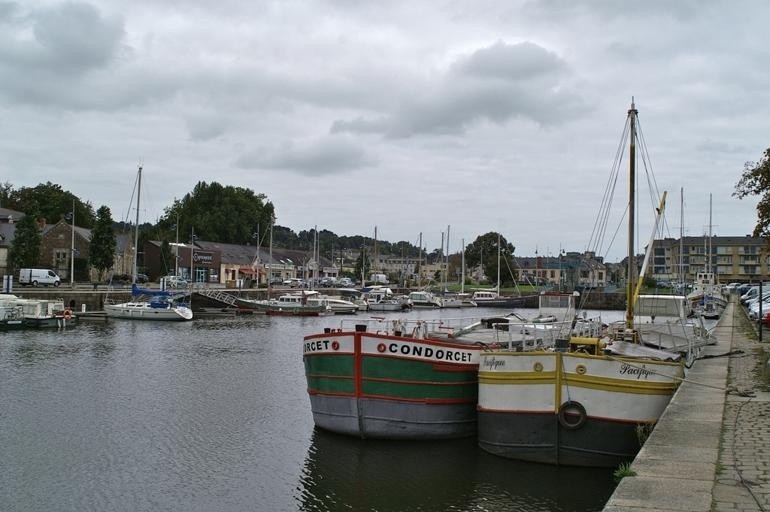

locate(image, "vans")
[[18, 268, 61, 287]]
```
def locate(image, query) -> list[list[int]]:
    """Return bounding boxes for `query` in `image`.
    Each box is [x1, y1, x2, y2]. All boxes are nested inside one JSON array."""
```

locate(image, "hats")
[[728, 282, 770, 330], [657, 281, 698, 289], [714, 284, 727, 290]]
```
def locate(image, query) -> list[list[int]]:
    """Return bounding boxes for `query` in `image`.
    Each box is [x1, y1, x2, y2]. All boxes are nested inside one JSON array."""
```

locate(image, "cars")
[[657, 281, 698, 289], [728, 282, 770, 330], [714, 284, 727, 290]]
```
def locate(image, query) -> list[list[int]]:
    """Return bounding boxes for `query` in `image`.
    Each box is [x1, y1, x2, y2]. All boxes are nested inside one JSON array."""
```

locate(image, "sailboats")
[[470, 230, 539, 310], [276, 225, 478, 313], [103, 167, 193, 321], [682, 192, 728, 320], [235, 212, 331, 317]]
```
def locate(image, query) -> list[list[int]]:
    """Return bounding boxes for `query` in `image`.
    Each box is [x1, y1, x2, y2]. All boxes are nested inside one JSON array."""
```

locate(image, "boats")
[[303, 310, 606, 441], [473, 95, 708, 469], [0, 294, 77, 331]]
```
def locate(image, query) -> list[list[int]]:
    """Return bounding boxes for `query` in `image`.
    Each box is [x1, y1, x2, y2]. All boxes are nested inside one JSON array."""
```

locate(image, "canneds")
[[63, 309, 72, 320], [558, 400, 587, 430]]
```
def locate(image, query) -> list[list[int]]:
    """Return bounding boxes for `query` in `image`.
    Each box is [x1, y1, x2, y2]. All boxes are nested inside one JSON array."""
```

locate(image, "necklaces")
[[682, 192, 728, 320]]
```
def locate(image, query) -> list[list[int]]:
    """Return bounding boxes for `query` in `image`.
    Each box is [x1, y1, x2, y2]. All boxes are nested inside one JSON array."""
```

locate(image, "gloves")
[[473, 95, 708, 469], [0, 294, 77, 331], [303, 310, 606, 441]]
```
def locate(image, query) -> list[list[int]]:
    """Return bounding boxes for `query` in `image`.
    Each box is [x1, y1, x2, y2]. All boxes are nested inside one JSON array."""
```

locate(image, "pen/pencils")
[[18, 268, 61, 287]]
